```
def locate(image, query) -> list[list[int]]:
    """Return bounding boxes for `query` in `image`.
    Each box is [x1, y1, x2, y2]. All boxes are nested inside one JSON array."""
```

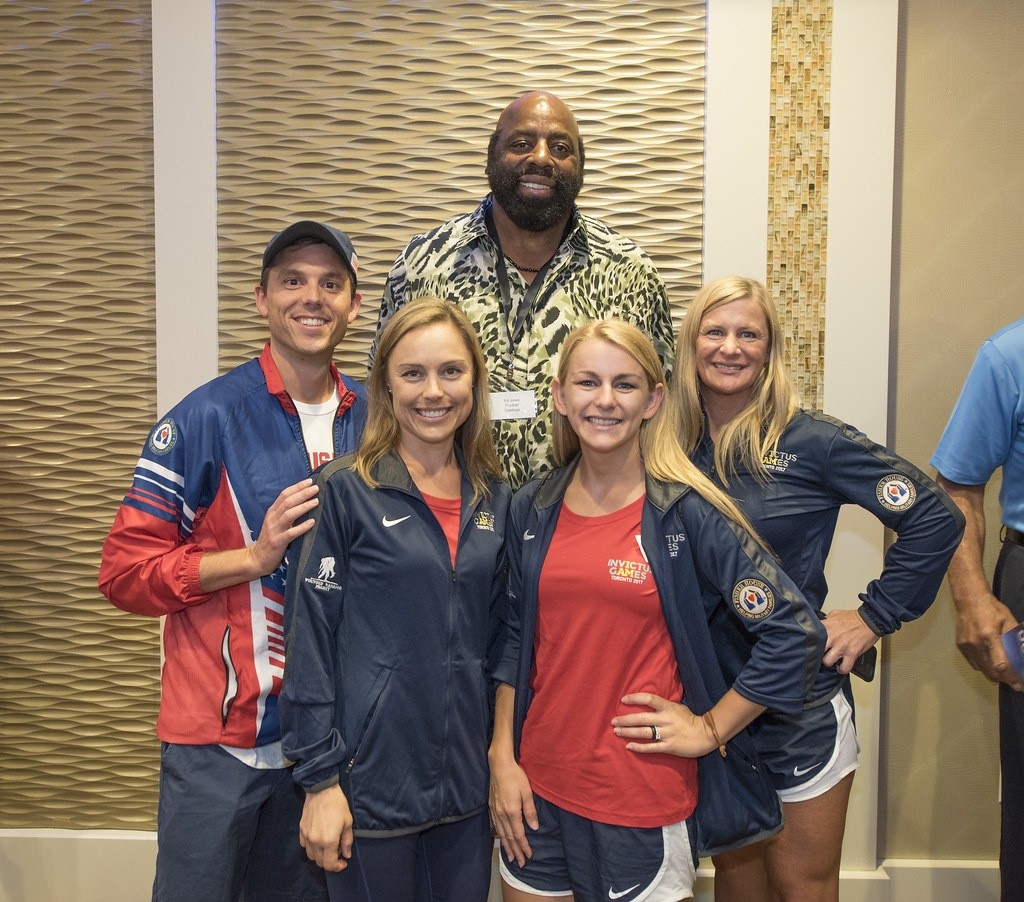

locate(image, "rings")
[[651, 726, 660, 740]]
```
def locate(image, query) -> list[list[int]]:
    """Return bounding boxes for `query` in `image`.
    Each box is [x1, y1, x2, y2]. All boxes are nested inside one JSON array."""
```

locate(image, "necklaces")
[[503, 253, 541, 272]]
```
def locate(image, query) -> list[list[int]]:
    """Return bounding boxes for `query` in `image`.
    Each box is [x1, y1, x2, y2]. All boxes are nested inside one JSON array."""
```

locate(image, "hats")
[[262, 221, 360, 296]]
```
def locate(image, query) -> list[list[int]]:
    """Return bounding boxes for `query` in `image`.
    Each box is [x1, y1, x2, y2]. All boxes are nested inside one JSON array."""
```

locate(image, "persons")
[[930, 316, 1024, 902], [278, 297, 513, 902], [365, 91, 675, 488], [99, 220, 371, 902], [487, 318, 828, 902], [668, 276, 967, 902]]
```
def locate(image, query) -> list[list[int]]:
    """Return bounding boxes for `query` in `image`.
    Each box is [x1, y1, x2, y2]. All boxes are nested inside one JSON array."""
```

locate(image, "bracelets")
[[705, 711, 727, 757]]
